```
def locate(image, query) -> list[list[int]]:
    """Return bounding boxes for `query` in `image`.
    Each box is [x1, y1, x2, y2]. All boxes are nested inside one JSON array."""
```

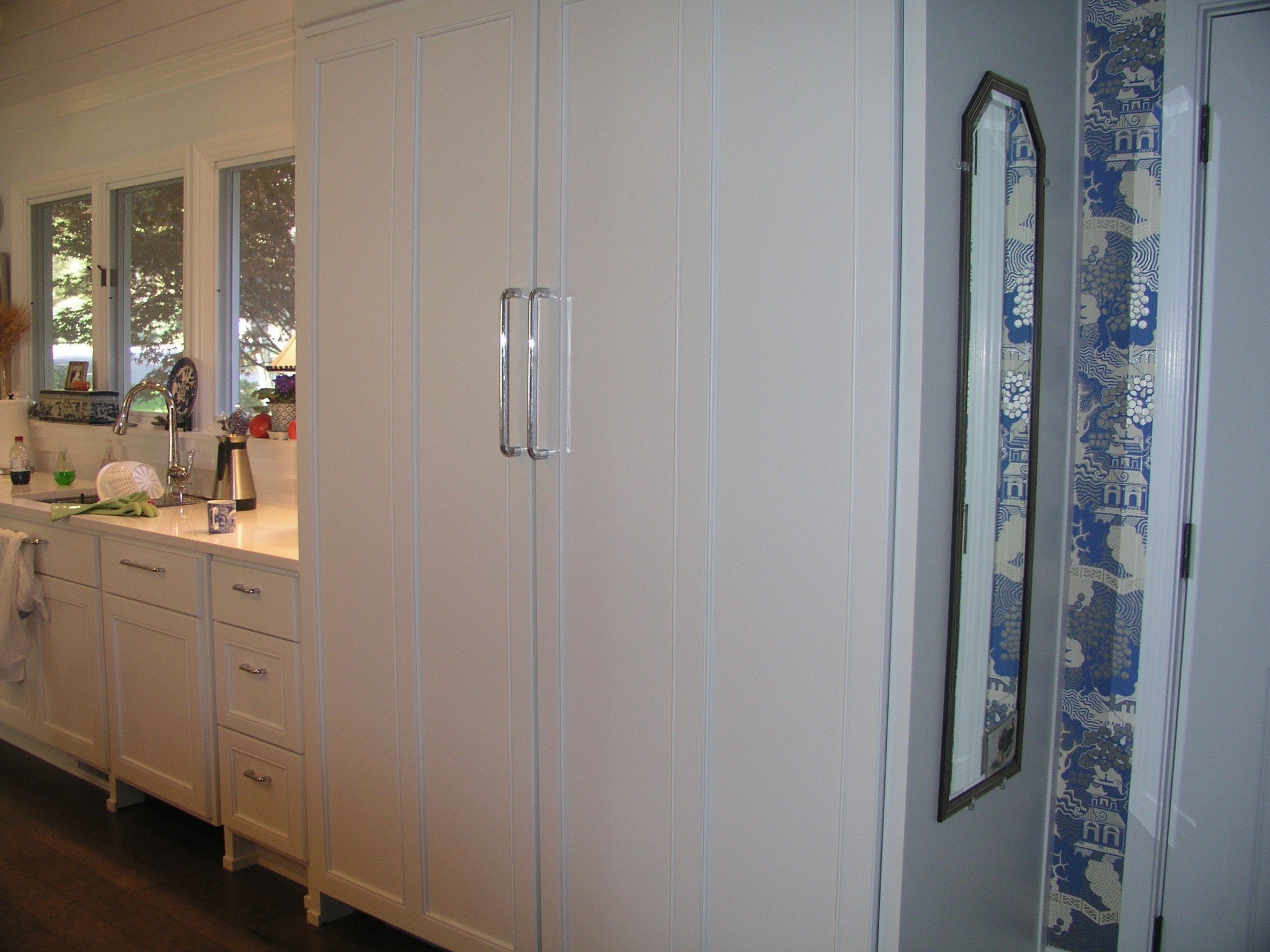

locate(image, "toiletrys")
[[52, 439, 76, 486]]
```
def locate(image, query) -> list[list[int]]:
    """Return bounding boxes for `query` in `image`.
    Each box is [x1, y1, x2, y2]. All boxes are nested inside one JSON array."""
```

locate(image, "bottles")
[[101, 440, 116, 470], [10, 435, 31, 486], [54, 441, 76, 486]]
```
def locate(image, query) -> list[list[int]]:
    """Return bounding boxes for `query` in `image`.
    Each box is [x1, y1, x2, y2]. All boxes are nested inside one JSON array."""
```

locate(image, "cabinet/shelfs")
[[1, 505, 309, 891], [290, 2, 1085, 952]]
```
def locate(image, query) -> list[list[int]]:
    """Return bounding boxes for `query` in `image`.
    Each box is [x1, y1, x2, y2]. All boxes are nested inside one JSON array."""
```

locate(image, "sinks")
[[12, 489, 199, 516]]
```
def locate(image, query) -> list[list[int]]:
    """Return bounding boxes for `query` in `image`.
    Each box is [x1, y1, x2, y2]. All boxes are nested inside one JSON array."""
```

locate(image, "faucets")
[[112, 380, 195, 498]]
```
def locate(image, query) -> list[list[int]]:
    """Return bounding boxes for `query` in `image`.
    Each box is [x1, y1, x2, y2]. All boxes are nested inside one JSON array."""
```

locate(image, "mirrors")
[[930, 68, 1047, 821]]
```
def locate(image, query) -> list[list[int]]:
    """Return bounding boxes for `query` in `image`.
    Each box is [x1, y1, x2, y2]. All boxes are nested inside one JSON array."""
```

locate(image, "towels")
[[0, 527, 53, 688]]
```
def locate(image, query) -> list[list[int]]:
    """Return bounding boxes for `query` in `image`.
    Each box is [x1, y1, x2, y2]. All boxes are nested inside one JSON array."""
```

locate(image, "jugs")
[[211, 433, 257, 513]]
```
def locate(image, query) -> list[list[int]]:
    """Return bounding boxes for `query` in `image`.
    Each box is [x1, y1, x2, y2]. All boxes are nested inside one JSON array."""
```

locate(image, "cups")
[[207, 499, 237, 534]]
